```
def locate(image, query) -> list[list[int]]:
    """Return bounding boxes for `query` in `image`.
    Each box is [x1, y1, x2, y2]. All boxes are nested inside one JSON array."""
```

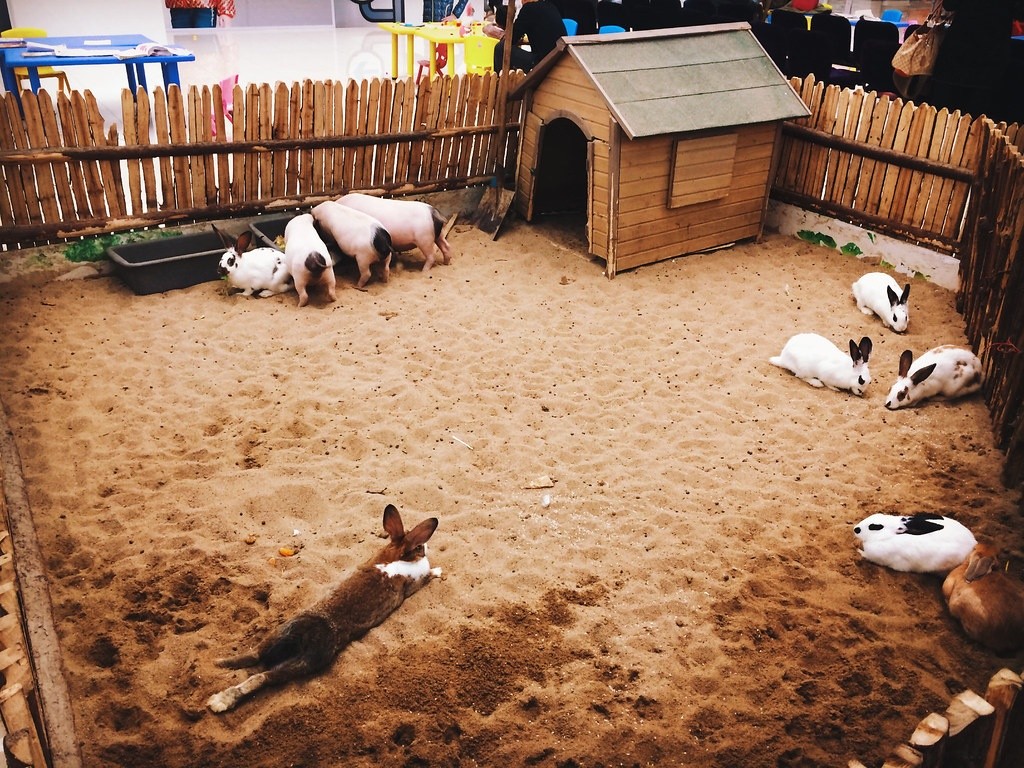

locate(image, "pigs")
[[284, 193, 451, 307]]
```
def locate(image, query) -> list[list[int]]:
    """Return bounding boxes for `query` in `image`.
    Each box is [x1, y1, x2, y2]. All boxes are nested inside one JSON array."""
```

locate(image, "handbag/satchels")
[[892, 0, 955, 77]]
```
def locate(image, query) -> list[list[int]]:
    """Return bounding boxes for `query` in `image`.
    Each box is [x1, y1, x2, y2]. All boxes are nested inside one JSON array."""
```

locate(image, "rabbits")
[[852, 272, 910, 332], [768, 332, 873, 396], [853, 512, 978, 573], [886, 345, 986, 409], [942, 543, 1024, 653], [207, 504, 443, 715], [211, 223, 295, 299]]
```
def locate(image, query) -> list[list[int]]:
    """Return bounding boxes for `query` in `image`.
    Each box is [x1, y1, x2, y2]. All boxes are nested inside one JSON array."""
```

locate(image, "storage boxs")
[[248, 215, 301, 255], [107, 228, 242, 296], [171, 6, 219, 46]]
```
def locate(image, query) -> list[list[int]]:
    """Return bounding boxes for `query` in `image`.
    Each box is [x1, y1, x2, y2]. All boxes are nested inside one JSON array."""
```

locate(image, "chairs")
[[464, 35, 500, 79], [1, 28, 72, 95]]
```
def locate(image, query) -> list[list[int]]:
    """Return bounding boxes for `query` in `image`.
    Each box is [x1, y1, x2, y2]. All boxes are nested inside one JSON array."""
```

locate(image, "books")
[[0, 37, 192, 61]]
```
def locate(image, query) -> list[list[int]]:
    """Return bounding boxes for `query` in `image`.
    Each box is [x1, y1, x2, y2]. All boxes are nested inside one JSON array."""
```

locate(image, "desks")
[[0, 34, 195, 128], [377, 21, 501, 97]]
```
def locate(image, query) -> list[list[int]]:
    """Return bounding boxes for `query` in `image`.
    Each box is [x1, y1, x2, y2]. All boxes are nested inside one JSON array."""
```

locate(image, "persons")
[[493, 0, 568, 75], [930, 0, 1024, 114]]
